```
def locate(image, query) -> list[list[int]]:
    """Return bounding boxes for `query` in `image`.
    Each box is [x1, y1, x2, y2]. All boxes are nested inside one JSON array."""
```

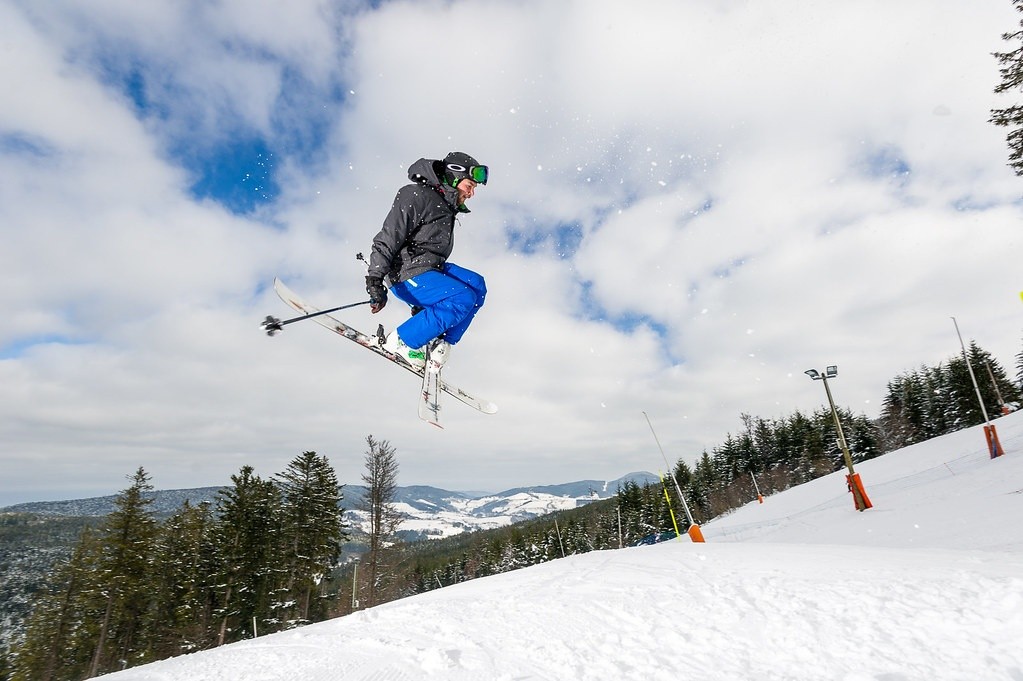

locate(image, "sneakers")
[[381, 328, 427, 371], [428, 338, 451, 374]]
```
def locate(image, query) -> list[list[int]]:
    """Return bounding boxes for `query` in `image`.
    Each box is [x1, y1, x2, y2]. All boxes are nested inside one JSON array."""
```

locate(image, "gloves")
[[365, 276, 388, 313], [411, 306, 424, 317]]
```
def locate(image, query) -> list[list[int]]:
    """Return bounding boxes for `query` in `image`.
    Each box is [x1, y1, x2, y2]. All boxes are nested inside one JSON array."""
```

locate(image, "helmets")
[[443, 151, 480, 187]]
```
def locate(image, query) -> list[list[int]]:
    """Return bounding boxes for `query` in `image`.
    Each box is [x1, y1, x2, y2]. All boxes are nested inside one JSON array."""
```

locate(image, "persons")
[[363, 151, 489, 378]]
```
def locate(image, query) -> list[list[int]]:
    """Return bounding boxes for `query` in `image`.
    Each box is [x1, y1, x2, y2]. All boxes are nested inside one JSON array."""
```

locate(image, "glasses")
[[469, 165, 488, 185]]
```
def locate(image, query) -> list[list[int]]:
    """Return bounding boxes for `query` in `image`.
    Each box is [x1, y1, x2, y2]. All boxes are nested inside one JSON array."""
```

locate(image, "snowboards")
[[272, 273, 502, 418]]
